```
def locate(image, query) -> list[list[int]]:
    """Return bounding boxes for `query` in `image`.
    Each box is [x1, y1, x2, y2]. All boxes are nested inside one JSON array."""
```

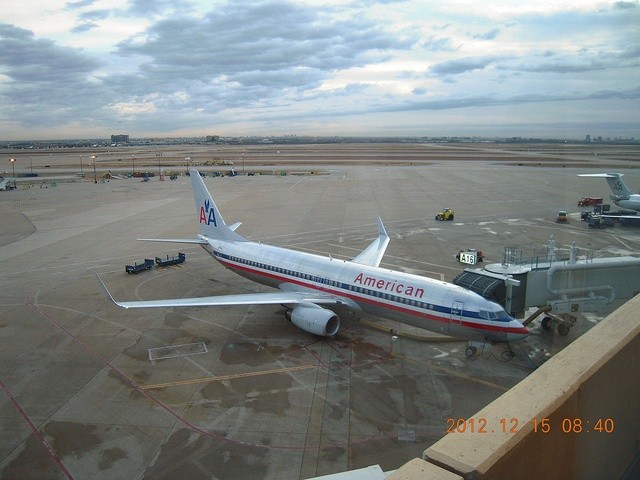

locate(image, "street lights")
[[131, 155, 135, 172], [156, 154, 162, 175], [9, 159, 16, 189], [90, 155, 97, 183], [185, 157, 190, 176]]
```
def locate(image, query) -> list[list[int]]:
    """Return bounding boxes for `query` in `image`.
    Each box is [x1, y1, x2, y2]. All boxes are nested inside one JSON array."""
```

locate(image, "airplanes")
[[94, 169, 529, 356], [577, 173, 640, 219]]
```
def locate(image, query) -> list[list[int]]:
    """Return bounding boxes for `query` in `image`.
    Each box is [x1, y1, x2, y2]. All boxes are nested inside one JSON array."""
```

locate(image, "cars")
[[557, 211, 567, 221]]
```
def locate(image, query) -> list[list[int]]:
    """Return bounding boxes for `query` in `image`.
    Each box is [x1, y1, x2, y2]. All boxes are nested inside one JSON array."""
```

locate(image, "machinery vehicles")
[[435, 208, 454, 221], [578, 198, 602, 206]]
